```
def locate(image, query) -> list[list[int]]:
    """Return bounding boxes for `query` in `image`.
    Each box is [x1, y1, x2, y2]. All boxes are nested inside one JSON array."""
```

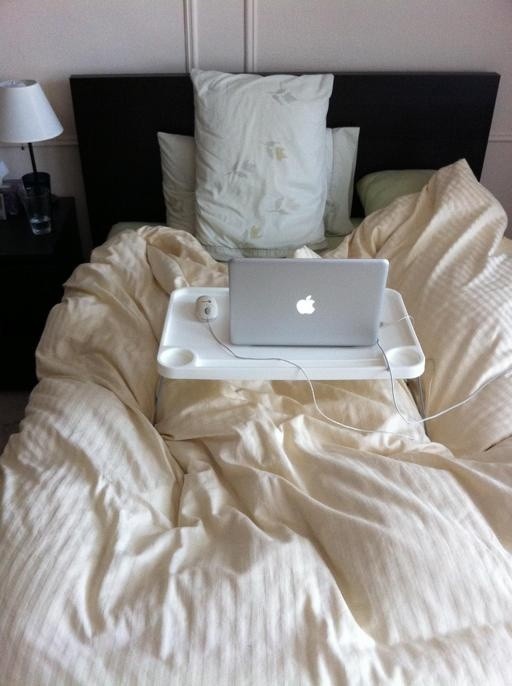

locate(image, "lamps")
[[0, 78, 65, 212]]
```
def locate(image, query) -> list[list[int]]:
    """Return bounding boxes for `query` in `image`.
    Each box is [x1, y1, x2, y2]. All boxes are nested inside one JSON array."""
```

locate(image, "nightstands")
[[0, 194, 87, 396]]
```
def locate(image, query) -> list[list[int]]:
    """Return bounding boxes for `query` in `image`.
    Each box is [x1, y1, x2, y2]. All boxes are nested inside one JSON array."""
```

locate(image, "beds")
[[0, 70, 511, 686]]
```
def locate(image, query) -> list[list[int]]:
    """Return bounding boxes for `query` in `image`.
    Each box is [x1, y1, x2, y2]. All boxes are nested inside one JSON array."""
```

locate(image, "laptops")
[[228, 258, 389, 347]]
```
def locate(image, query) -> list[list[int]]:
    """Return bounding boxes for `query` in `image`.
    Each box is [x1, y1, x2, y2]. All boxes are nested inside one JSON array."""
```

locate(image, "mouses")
[[196, 295, 217, 322]]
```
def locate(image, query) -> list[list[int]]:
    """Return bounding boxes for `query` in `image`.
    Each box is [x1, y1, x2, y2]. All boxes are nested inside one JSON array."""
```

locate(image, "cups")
[[19, 183, 52, 235]]
